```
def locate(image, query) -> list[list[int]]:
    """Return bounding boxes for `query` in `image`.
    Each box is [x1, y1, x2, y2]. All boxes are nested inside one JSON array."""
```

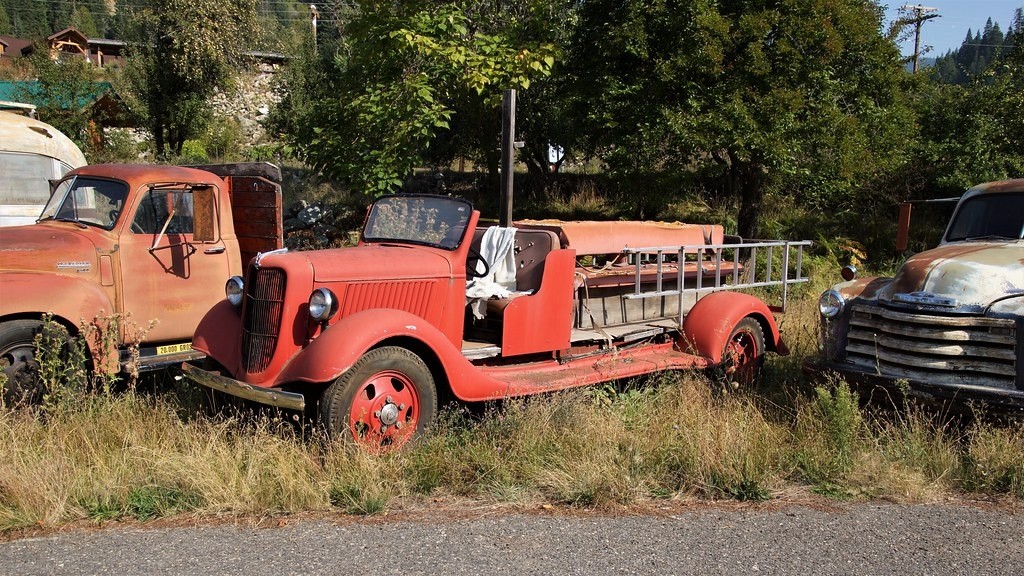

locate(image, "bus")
[[0, 100, 103, 229]]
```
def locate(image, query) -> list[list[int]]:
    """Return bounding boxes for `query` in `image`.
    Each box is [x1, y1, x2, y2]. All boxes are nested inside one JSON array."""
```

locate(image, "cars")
[[181, 194, 816, 465]]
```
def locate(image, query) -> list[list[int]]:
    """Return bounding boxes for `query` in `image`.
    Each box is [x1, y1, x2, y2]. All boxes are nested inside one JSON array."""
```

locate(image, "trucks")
[[0, 162, 286, 415]]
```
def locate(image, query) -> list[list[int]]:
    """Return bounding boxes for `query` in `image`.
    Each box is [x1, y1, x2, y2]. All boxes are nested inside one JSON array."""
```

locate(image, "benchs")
[[444, 225, 559, 340], [518, 220, 746, 288]]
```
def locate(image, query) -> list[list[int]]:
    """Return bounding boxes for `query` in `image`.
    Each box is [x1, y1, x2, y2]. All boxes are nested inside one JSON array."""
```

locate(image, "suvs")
[[801, 178, 1023, 426]]
